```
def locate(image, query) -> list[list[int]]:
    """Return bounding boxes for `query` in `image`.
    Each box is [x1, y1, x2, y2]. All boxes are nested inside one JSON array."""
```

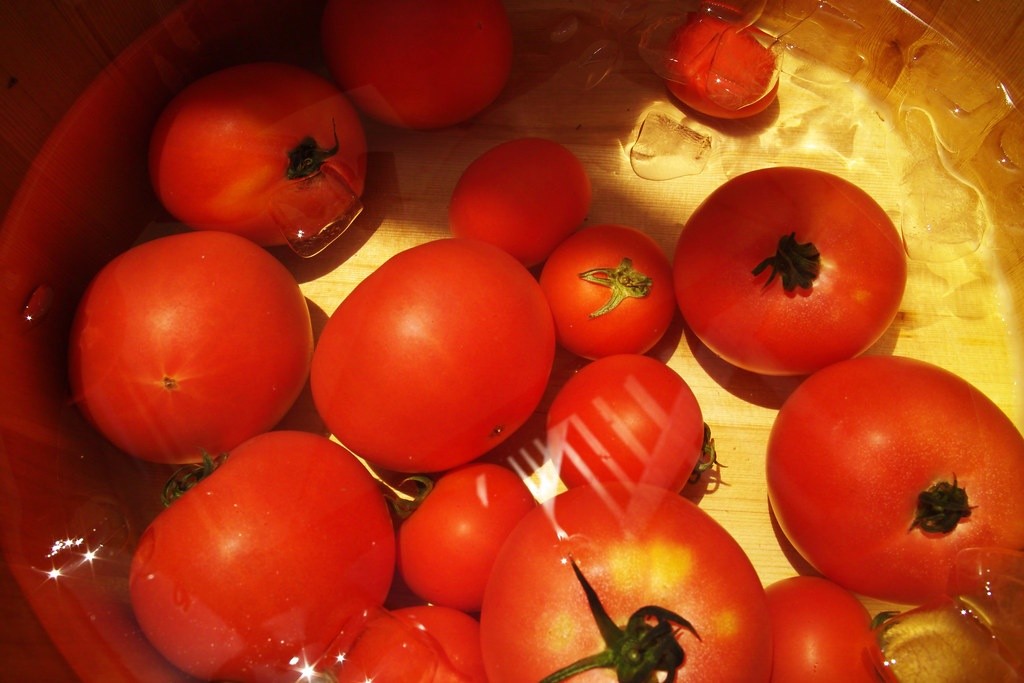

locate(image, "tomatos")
[[68, 0, 1024, 683]]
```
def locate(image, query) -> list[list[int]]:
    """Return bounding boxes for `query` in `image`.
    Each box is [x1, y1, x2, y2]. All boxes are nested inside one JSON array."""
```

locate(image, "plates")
[[0, 0, 1024, 683]]
